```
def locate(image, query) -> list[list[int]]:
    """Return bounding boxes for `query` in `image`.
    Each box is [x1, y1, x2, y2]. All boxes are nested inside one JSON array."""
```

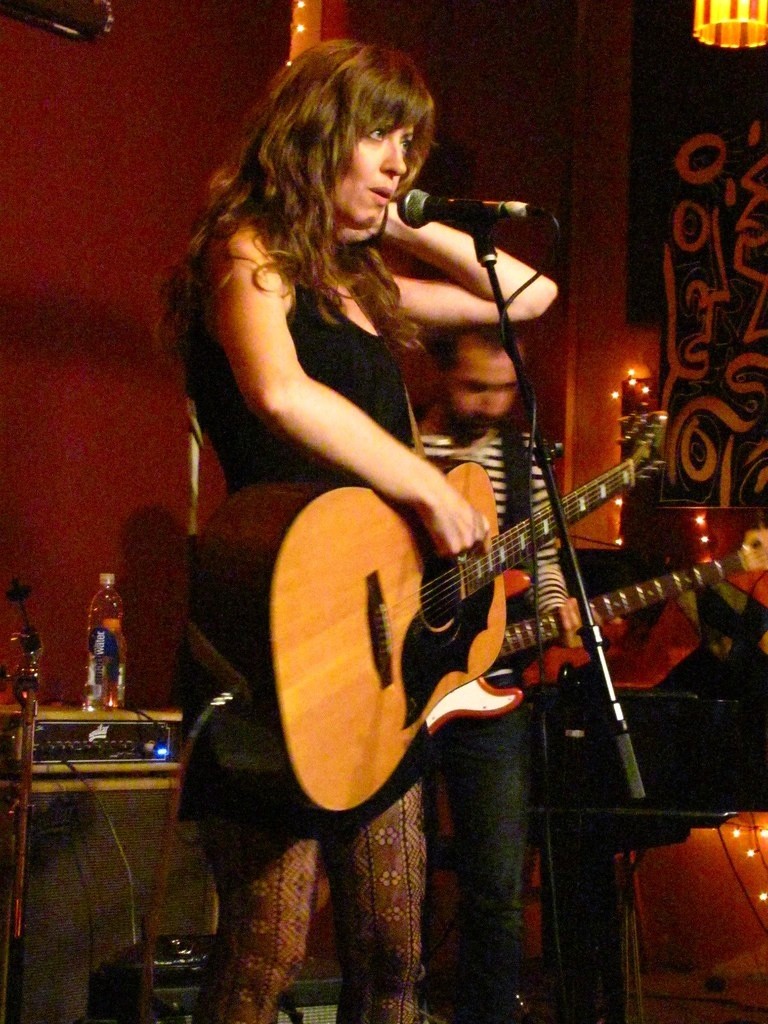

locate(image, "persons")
[[406, 325, 588, 1024], [160, 36, 561, 1024]]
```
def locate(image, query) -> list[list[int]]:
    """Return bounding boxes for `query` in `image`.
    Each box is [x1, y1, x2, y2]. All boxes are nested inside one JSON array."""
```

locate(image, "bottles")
[[83, 572, 124, 710]]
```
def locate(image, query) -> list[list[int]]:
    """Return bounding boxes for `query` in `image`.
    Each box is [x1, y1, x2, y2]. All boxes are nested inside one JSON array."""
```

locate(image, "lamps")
[[692, 0, 768, 51]]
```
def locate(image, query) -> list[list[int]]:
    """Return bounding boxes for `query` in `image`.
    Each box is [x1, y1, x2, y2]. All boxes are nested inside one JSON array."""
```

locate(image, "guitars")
[[425, 543, 767, 734], [192, 409, 670, 813]]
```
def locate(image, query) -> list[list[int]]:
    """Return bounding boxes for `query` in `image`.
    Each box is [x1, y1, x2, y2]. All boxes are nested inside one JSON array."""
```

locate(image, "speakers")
[[522, 692, 740, 823], [0, 705, 224, 1024]]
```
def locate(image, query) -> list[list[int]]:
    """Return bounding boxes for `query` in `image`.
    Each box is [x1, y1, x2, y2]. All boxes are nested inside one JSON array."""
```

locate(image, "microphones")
[[398, 189, 551, 230]]
